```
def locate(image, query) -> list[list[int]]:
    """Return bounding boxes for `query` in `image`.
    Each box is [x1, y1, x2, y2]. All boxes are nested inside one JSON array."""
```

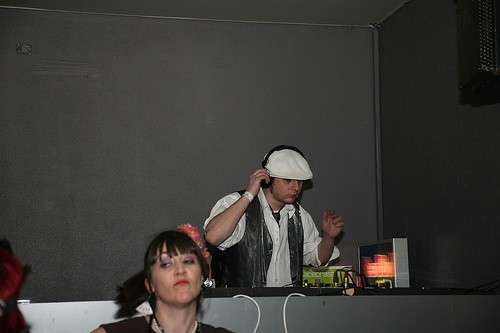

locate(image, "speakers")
[[456, 0, 500, 103]]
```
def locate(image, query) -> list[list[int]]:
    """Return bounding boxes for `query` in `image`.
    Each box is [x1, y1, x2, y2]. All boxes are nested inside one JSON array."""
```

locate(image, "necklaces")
[[152, 317, 198, 333]]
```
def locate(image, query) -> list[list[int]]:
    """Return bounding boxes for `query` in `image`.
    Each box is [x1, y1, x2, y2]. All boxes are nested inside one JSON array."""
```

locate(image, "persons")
[[90, 230, 236, 333], [203, 145, 344, 287]]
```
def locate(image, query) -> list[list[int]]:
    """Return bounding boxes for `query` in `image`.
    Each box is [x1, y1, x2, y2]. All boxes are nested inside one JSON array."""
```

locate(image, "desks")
[[17, 294, 500, 333]]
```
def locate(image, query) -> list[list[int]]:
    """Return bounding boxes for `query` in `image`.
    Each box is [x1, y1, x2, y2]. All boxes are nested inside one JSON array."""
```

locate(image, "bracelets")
[[243, 191, 254, 203]]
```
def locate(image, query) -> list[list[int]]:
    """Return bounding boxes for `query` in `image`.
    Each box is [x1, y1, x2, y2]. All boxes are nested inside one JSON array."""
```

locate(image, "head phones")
[[260, 145, 305, 188]]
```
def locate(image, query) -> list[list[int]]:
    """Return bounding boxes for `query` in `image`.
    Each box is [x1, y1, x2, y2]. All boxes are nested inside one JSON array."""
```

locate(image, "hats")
[[264, 149, 313, 181]]
